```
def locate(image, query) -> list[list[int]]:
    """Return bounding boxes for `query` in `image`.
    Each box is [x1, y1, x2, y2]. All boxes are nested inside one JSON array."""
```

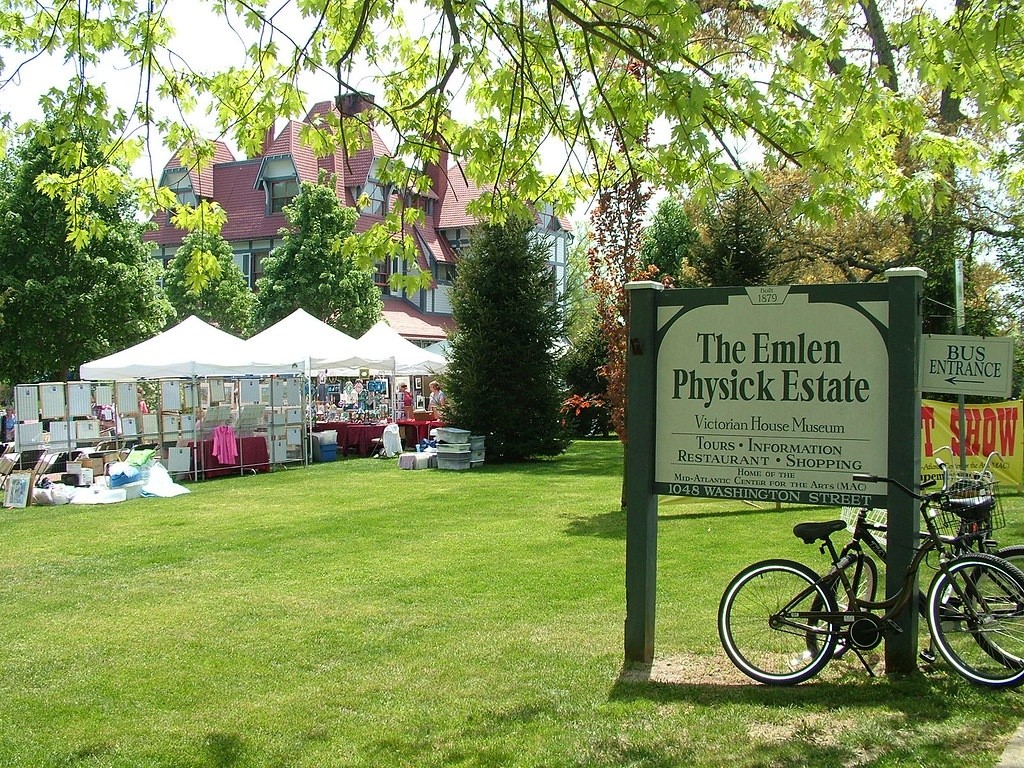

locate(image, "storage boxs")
[[399, 427, 485, 470], [62, 450, 131, 487], [318, 442, 338, 462]]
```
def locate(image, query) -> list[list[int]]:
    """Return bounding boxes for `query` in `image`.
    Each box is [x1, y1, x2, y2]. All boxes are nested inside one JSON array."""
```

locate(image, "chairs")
[[369, 423, 397, 458]]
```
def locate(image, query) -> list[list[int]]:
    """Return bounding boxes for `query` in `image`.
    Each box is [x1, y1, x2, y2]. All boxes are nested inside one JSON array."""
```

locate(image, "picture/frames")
[[5, 474, 30, 508]]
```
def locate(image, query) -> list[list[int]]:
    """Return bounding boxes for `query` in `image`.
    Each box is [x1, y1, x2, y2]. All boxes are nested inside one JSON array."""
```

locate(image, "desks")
[[184, 436, 269, 479], [312, 418, 444, 455]]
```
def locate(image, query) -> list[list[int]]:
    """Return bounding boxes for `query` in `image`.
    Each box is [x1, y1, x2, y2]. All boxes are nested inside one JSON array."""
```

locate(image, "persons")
[[427, 381, 445, 423], [137, 385, 239, 443], [84, 385, 123, 439], [0, 405, 18, 444], [396, 381, 414, 407]]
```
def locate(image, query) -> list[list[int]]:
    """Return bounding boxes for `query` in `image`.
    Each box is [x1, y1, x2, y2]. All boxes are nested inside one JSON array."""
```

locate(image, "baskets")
[[840, 506, 888, 546], [930, 480, 1006, 535]]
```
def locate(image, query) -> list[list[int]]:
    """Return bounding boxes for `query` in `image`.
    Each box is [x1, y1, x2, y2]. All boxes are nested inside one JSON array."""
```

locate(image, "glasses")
[[401, 385, 407, 388]]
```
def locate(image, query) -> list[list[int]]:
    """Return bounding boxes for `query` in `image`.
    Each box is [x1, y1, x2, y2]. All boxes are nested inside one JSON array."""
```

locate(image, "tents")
[[245, 308, 461, 464], [79, 315, 312, 483]]
